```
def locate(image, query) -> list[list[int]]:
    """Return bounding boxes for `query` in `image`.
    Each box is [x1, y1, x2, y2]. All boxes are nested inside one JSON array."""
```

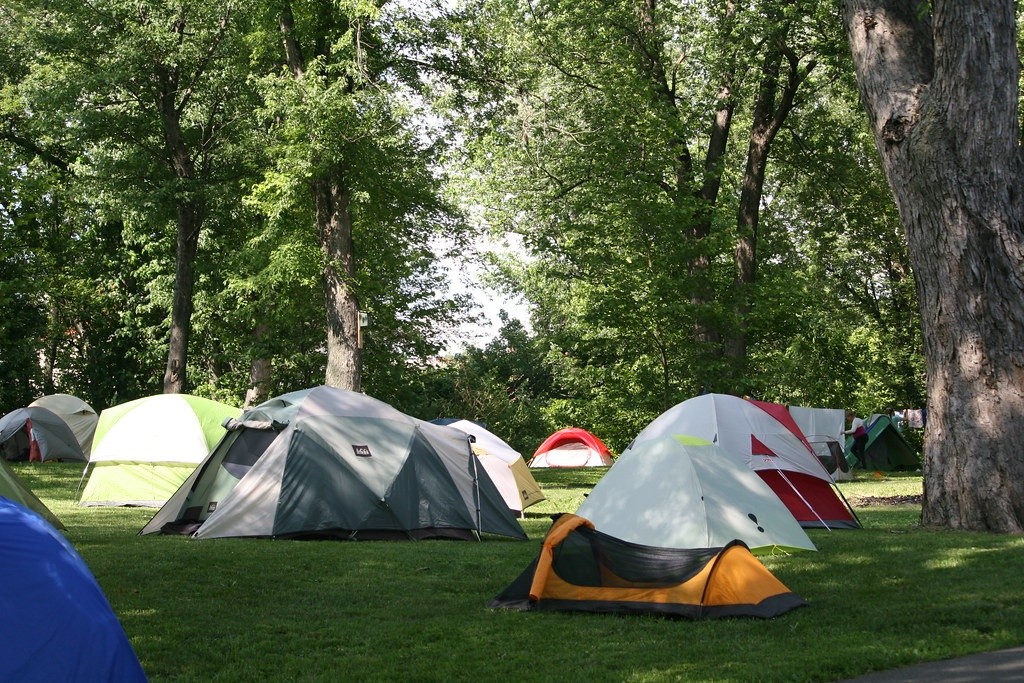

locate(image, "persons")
[[840, 412, 869, 469]]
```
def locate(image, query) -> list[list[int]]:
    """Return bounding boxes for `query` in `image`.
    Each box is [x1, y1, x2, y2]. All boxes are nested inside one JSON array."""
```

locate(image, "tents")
[[426, 418, 547, 520], [0, 494, 148, 683], [484, 511, 816, 622], [135, 385, 532, 543], [27, 393, 96, 462], [526, 428, 619, 469], [611, 393, 866, 532], [801, 435, 855, 482], [75, 392, 245, 508], [845, 414, 922, 473], [0, 457, 71, 533], [571, 436, 818, 556], [0, 407, 88, 463]]
